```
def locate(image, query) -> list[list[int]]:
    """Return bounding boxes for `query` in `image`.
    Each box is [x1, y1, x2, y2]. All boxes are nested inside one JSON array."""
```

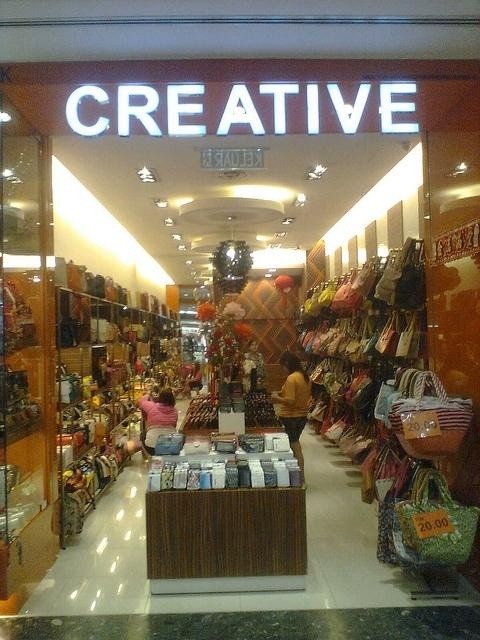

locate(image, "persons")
[[267, 351, 314, 489], [242, 340, 268, 393], [134, 387, 179, 463], [184, 360, 203, 392]]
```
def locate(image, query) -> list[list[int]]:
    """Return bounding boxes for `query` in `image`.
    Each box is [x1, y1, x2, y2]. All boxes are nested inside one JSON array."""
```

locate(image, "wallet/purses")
[[146, 458, 303, 493], [210, 433, 290, 453]]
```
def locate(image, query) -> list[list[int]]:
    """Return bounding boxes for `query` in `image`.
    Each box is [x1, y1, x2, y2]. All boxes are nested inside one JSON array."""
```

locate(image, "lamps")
[[209, 217, 254, 296]]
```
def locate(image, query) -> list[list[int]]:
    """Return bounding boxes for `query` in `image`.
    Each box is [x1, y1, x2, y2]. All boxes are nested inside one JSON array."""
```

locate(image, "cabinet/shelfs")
[[145, 441, 308, 596], [56, 283, 184, 550], [0, 96, 62, 616]]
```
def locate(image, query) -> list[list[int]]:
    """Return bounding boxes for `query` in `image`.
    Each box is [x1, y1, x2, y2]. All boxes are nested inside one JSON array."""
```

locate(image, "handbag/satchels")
[[360, 379, 479, 569], [3, 278, 40, 356], [55, 338, 184, 535], [56, 257, 182, 348], [297, 237, 425, 469], [0, 366, 41, 432]]
